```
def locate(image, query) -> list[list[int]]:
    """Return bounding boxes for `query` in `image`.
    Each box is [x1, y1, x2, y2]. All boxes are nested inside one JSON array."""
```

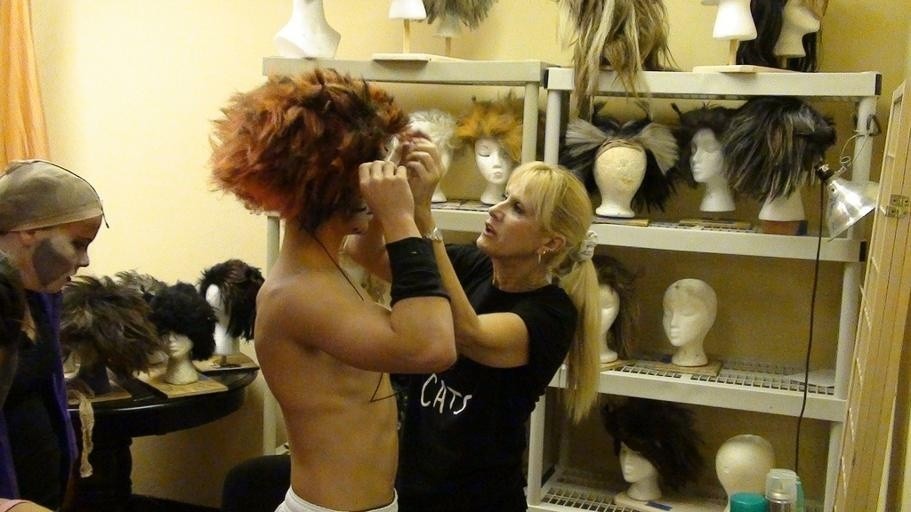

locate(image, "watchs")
[[425, 226, 443, 242]]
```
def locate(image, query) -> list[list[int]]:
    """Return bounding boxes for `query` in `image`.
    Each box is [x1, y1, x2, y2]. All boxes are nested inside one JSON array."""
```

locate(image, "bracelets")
[[382, 236, 452, 311]]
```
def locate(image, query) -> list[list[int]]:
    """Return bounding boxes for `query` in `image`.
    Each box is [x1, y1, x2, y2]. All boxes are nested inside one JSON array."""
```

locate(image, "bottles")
[[728, 466, 807, 512]]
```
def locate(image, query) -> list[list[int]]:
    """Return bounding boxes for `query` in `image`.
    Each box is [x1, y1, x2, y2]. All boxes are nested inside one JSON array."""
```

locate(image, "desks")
[[55, 353, 258, 510]]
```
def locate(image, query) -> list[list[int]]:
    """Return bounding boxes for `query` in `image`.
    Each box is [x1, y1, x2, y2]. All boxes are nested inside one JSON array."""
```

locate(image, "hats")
[[1, 159, 109, 233]]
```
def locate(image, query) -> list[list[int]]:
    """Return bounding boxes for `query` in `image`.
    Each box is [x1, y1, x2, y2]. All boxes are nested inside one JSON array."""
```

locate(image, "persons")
[[660, 279, 718, 368], [0, 154, 109, 512], [587, 253, 638, 363], [143, 280, 220, 388], [341, 125, 607, 512], [600, 399, 703, 503], [714, 434, 775, 512], [201, 65, 462, 512], [0, 251, 67, 512], [401, 0, 835, 225], [58, 276, 158, 396], [193, 257, 269, 364]]
